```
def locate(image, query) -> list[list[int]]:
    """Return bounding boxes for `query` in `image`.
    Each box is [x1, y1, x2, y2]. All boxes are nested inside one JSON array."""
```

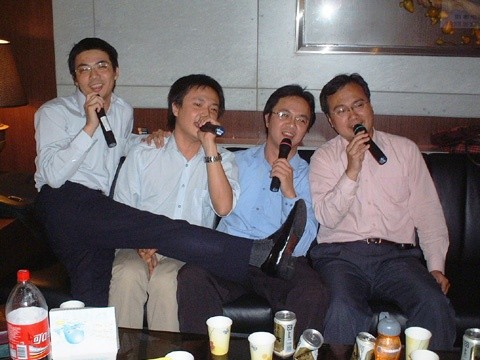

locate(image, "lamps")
[[0, 39, 29, 155]]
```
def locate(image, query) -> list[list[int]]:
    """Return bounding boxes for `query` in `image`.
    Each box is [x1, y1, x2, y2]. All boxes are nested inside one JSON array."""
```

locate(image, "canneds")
[[292, 329, 324, 360], [350, 331, 376, 360], [273, 310, 297, 357], [461, 328, 480, 360]]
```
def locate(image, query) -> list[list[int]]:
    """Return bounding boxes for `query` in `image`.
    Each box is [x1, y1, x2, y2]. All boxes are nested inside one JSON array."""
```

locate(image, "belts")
[[361, 238, 414, 246]]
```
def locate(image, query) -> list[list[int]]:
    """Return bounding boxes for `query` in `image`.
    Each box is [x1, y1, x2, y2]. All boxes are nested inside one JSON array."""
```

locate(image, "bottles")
[[374, 312, 402, 360], [5, 270, 51, 360]]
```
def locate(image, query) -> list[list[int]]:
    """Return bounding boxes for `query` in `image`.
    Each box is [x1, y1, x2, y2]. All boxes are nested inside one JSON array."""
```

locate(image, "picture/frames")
[[295, 0, 480, 57]]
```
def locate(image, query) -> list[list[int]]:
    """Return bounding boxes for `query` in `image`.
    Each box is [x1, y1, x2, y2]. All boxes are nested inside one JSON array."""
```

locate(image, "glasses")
[[329, 101, 370, 118], [271, 111, 308, 128], [75, 62, 113, 73]]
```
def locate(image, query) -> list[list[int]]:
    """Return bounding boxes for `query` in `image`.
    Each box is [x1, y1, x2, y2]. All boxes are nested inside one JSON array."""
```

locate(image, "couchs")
[[29, 147, 480, 336]]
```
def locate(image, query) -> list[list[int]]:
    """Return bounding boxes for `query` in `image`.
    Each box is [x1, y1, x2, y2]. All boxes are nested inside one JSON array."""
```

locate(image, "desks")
[[116, 325, 462, 360]]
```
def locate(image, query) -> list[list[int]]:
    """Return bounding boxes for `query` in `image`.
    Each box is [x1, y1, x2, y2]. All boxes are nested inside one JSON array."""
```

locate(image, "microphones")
[[270, 138, 291, 192], [95, 94, 117, 148], [353, 124, 387, 165], [200, 122, 224, 136]]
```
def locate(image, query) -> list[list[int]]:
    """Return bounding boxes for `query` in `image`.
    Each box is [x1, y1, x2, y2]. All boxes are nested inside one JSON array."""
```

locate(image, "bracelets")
[[204, 152, 222, 163]]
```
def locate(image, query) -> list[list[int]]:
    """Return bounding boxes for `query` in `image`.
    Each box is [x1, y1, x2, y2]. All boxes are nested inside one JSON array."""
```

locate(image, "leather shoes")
[[260, 199, 307, 282]]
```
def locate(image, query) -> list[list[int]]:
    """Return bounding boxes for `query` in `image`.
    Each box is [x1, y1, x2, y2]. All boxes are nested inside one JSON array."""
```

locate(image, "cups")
[[206, 316, 233, 355], [166, 351, 195, 360], [404, 327, 432, 360], [60, 300, 85, 309], [410, 350, 439, 360], [248, 332, 276, 360]]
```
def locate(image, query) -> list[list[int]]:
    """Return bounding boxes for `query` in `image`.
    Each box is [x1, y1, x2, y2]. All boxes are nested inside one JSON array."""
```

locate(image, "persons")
[[176, 84, 329, 343], [34, 38, 307, 308], [107, 73, 241, 332], [309, 73, 458, 360]]
